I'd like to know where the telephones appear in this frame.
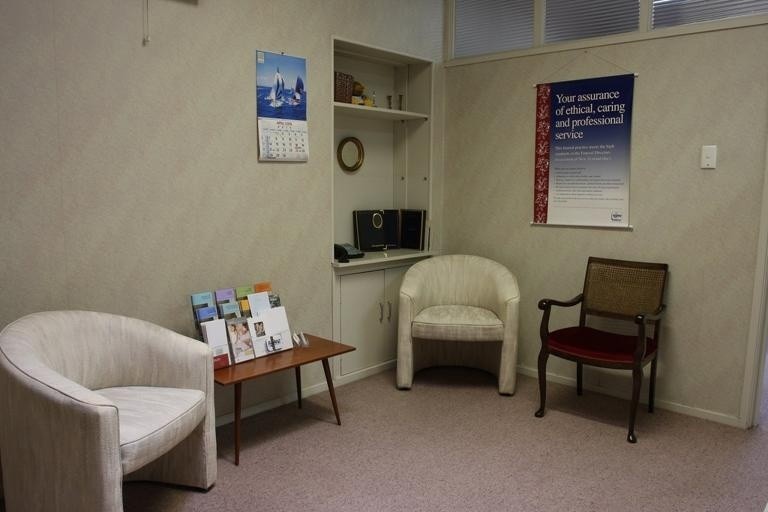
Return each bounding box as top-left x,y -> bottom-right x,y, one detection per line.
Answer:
333,242 -> 364,259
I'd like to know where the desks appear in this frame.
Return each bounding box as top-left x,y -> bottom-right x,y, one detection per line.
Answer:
214,334 -> 357,467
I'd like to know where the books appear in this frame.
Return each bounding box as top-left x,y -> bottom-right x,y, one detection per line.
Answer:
191,281 -> 294,372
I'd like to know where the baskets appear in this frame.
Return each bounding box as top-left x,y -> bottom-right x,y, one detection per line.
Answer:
334,71 -> 352,104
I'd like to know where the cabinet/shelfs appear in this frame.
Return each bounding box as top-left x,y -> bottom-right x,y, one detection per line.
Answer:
331,34 -> 434,385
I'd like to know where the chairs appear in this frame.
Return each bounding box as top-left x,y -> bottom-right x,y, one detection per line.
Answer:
535,254 -> 669,443
395,253 -> 520,395
0,310 -> 218,512
